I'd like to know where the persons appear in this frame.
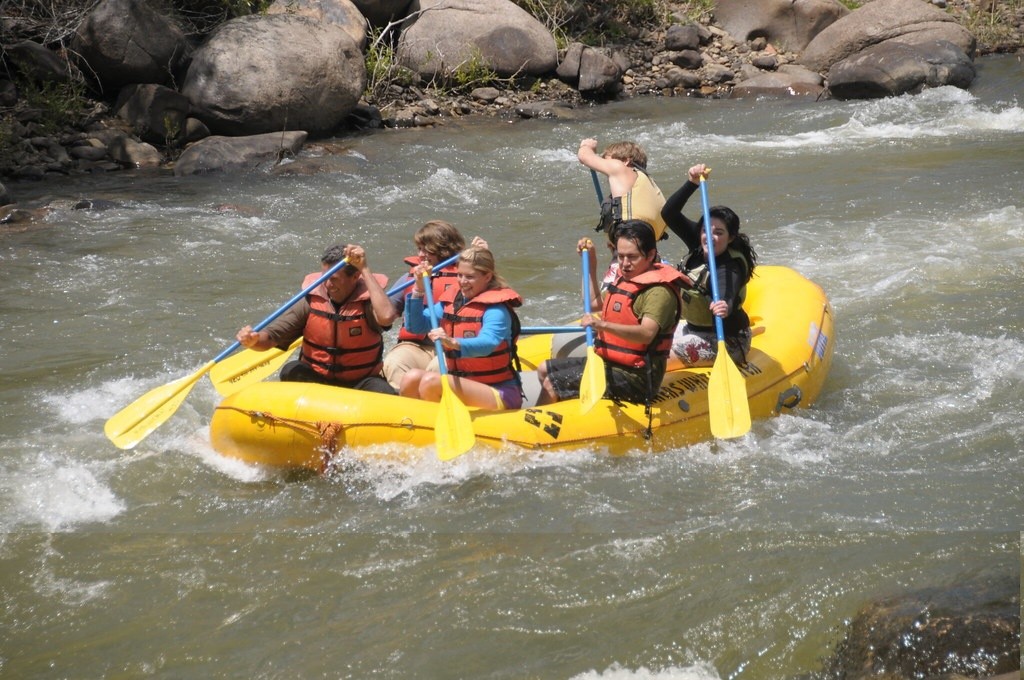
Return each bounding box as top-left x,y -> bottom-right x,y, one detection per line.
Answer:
400,246 -> 523,410
578,138 -> 667,292
236,243 -> 396,394
380,219 -> 488,392
535,217 -> 694,407
661,164 -> 760,372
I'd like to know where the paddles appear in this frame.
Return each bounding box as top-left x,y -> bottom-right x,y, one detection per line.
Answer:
588,136 -> 606,207
208,250 -> 463,398
103,254 -> 351,451
700,169 -> 754,440
515,324 -> 588,336
578,239 -> 608,417
421,269 -> 476,462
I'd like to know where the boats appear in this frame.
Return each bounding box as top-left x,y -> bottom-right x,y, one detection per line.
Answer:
210,265 -> 834,476
